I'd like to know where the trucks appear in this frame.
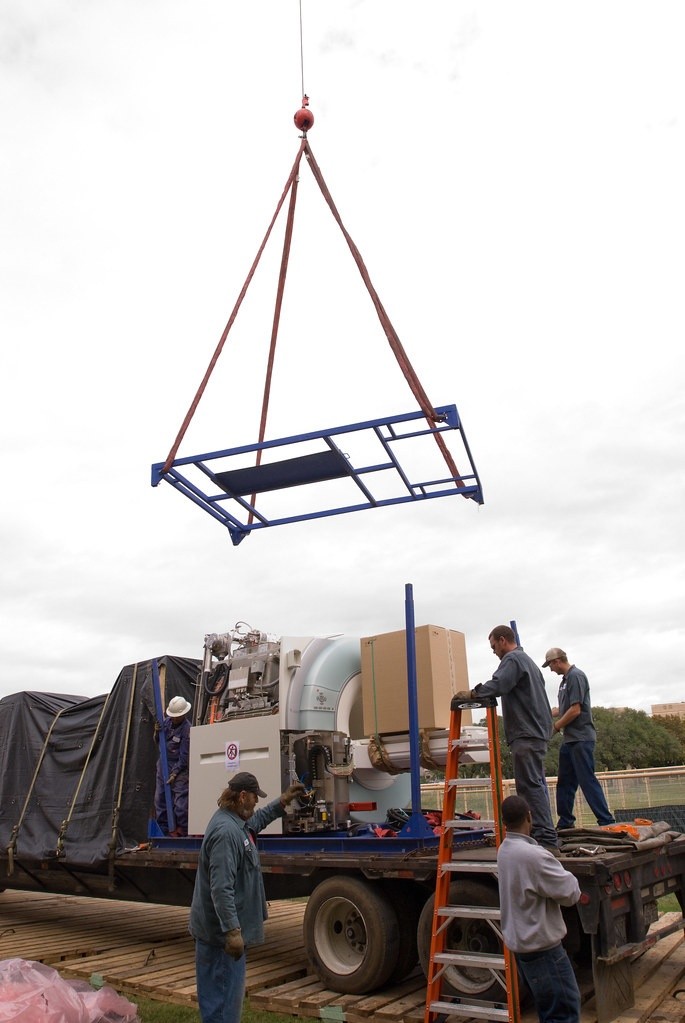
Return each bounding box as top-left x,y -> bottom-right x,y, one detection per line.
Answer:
1,622 -> 684,996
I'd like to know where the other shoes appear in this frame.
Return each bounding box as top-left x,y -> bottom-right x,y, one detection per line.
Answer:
544,847 -> 562,857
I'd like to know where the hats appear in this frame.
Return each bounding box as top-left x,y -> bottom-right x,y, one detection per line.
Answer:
228,771 -> 267,799
542,648 -> 566,668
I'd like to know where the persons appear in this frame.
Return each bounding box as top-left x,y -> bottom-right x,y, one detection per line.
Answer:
153,696 -> 191,836
542,648 -> 615,829
452,625 -> 561,857
496,795 -> 581,1023
187,773 -> 304,1023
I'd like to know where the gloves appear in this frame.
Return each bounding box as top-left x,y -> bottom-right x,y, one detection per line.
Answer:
453,690 -> 473,700
154,721 -> 162,735
166,773 -> 177,784
551,723 -> 559,739
225,928 -> 244,962
279,783 -> 305,807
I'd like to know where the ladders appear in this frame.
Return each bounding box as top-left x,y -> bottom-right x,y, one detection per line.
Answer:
422,694 -> 525,1023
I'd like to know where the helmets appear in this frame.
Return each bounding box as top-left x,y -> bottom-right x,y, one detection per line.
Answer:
165,696 -> 191,717
